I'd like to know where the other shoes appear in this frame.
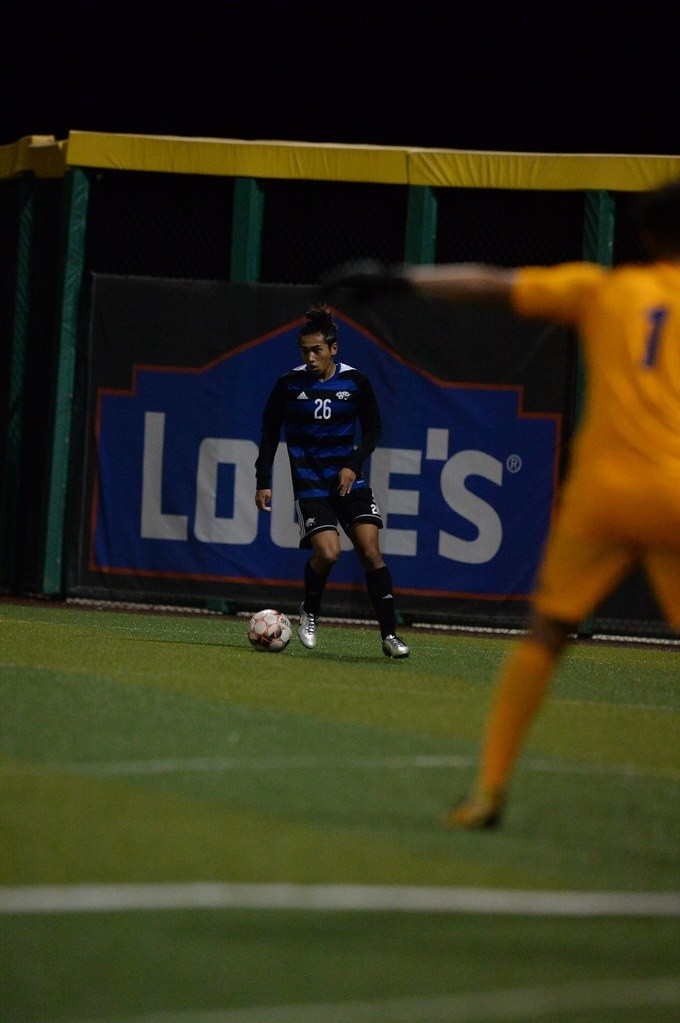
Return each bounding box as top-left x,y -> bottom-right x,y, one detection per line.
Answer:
444,779 -> 507,830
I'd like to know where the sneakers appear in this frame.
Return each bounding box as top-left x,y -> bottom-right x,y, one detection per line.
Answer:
382,633 -> 410,658
298,601 -> 319,649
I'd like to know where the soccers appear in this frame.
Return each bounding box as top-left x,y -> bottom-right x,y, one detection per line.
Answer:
246,607 -> 294,652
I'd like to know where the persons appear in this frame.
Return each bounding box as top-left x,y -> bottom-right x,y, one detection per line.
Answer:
407,182 -> 680,833
253,307 -> 410,659
